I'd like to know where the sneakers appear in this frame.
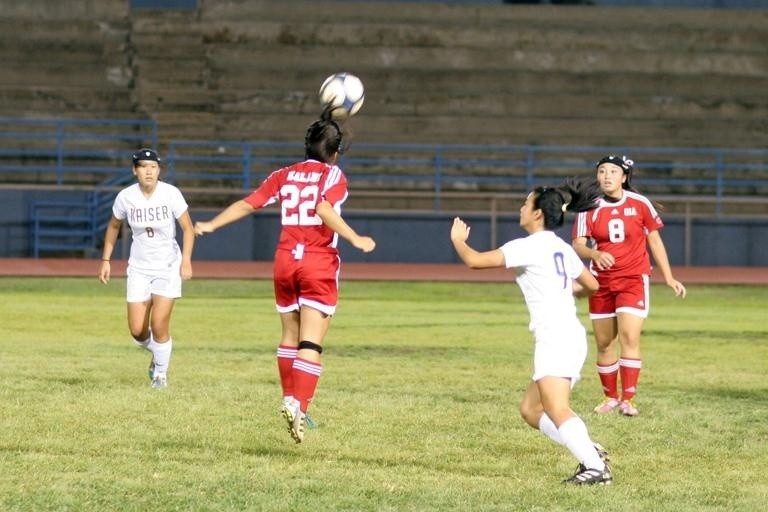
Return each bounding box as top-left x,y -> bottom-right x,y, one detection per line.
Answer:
593,396 -> 620,415
560,444 -> 613,488
149,353 -> 166,389
618,399 -> 639,417
281,398 -> 316,444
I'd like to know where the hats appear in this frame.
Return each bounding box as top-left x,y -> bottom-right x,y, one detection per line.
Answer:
596,154 -> 630,172
131,148 -> 161,163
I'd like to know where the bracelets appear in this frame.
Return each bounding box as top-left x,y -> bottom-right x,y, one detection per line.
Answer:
100,257 -> 112,263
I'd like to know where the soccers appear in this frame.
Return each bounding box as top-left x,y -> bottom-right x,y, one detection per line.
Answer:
319,72 -> 364,118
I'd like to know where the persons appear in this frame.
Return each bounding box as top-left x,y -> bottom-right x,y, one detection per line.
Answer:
98,147 -> 197,384
450,172 -> 612,484
570,153 -> 689,414
190,105 -> 375,446
